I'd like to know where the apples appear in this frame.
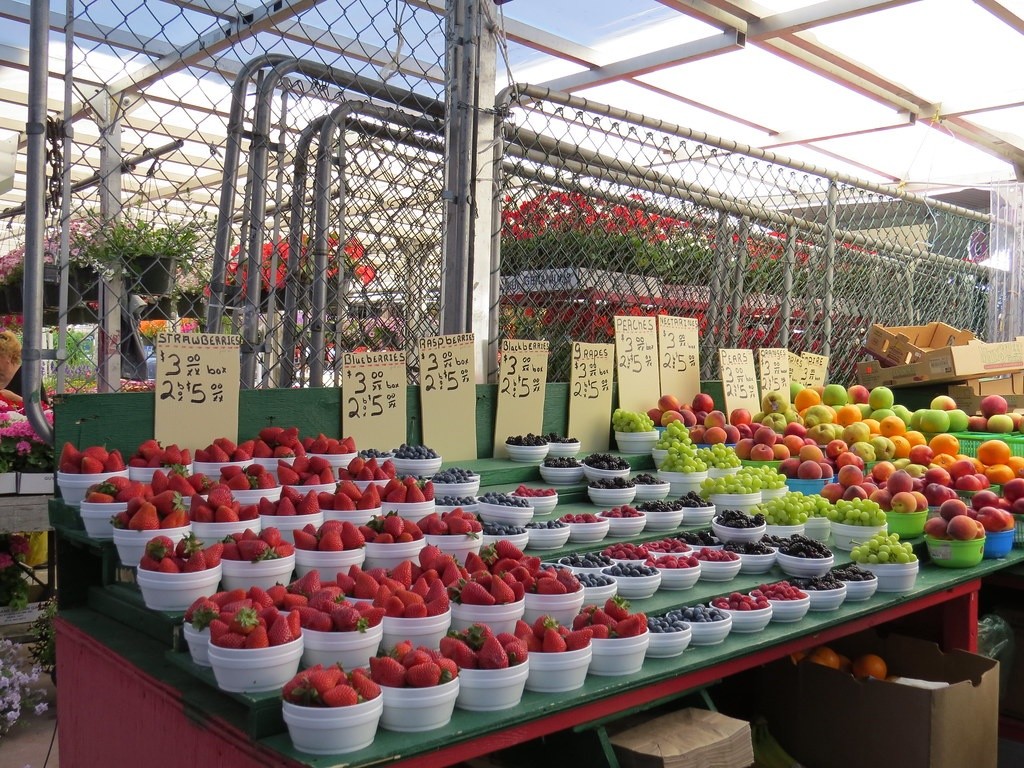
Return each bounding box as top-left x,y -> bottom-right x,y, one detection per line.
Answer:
787,382 -> 1024,434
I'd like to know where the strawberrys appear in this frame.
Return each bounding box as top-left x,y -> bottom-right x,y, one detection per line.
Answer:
59,425 -> 647,708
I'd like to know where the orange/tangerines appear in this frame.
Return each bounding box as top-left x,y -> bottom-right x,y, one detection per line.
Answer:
793,389 -> 1024,483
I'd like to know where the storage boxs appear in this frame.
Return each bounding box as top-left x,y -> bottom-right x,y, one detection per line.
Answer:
499,266 -> 864,311
766,632 -> 1001,767
857,322 -> 1024,415
0,582 -> 49,627
0,472 -> 54,494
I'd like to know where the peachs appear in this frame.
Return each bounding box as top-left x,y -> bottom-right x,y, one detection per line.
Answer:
735,422 -> 986,540
963,478 -> 1024,533
645,394 -> 763,445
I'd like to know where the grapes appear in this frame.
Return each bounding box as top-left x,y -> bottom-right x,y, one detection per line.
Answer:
612,409 -> 918,565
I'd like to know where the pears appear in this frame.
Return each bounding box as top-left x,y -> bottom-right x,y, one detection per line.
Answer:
752,391 -> 944,478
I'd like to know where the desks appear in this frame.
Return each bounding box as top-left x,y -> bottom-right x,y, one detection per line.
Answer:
49,381 -> 1024,768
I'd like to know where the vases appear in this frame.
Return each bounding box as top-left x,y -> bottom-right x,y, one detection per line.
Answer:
143,345 -> 155,379
0,254 -> 381,324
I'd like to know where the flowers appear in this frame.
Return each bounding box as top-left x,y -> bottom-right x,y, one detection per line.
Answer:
497,189 -> 877,298
0,532 -> 49,611
0,394 -> 55,477
0,198 -> 378,300
138,311 -> 406,351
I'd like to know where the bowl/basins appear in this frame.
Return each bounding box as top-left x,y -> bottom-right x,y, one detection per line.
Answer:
588,481 -> 671,507
57,451 -> 649,754
654,422 -> 1016,569
507,491 -> 558,515
558,515 -> 878,658
615,428 -> 919,593
556,505 -> 716,543
504,441 -> 580,461
539,461 -> 631,485
